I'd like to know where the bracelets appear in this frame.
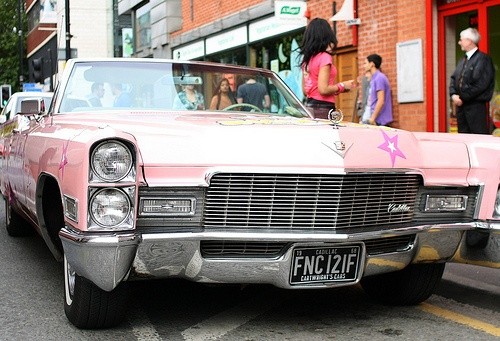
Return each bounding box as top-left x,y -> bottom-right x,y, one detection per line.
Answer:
334,82 -> 345,95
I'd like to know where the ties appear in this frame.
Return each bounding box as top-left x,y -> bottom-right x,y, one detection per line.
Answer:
457,55 -> 468,92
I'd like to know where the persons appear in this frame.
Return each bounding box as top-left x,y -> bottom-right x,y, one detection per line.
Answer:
363,53 -> 393,126
173,72 -> 206,110
235,75 -> 270,112
359,71 -> 373,125
449,27 -> 496,135
209,78 -> 238,111
110,83 -> 137,108
291,17 -> 356,120
85,82 -> 106,108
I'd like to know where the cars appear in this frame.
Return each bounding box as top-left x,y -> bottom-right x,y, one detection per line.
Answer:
1,92 -> 93,126
0,57 -> 500,330
